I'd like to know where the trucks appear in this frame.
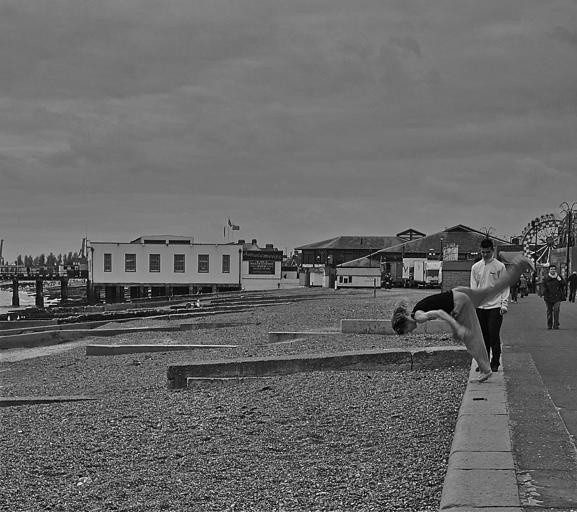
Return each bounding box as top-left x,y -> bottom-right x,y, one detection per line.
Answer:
402,257 -> 442,289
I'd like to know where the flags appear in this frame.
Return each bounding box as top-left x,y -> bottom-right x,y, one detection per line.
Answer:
223,217 -> 233,238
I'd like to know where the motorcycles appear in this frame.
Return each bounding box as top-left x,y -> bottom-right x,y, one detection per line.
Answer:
384,274 -> 393,289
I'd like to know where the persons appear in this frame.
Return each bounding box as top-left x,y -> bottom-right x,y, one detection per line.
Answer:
511,264 -> 577,305
470,239 -> 511,375
381,270 -> 393,290
390,255 -> 537,383
539,265 -> 566,330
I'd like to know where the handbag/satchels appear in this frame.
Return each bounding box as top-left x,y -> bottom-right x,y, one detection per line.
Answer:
560,285 -> 567,301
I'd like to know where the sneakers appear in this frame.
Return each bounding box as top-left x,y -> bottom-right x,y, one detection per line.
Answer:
474,363 -> 499,382
517,254 -> 536,272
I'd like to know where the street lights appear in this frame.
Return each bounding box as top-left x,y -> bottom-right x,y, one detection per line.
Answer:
559,201 -> 577,302
86,246 -> 95,300
